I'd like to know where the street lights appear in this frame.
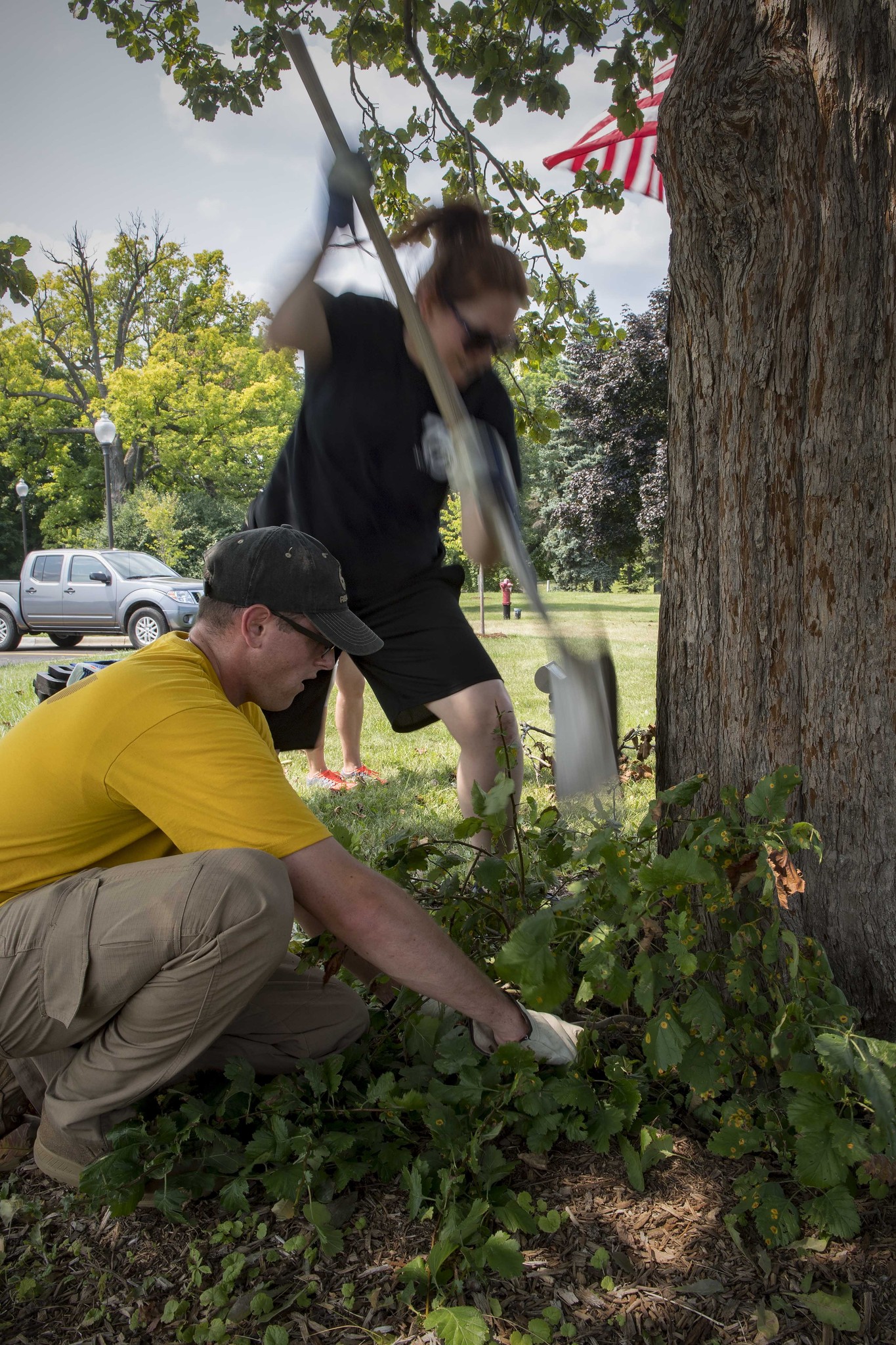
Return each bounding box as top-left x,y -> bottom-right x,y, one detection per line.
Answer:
94,409 -> 122,549
15,477 -> 30,559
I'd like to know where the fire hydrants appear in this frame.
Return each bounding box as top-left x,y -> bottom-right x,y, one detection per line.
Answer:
498,579 -> 514,620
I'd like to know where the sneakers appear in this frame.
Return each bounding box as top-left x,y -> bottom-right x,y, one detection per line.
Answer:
304,770 -> 356,792
342,767 -> 389,788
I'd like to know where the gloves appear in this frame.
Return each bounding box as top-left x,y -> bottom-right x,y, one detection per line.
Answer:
466,989 -> 586,1064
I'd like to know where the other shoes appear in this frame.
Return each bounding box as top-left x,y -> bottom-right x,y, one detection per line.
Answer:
33,1104 -> 113,1183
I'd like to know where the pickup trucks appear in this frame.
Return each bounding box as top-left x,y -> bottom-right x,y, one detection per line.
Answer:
0,548 -> 205,650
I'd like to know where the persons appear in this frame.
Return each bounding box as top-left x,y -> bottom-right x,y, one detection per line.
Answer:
0,524 -> 583,1188
255,152 -> 527,873
302,649 -> 389,790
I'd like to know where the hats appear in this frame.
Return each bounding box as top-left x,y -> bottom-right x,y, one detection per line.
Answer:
204,523 -> 385,656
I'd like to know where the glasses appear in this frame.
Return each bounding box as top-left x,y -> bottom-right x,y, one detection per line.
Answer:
438,288 -> 520,354
266,607 -> 336,659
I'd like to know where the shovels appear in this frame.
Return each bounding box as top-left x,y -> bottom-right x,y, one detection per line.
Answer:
275,23 -> 624,807
